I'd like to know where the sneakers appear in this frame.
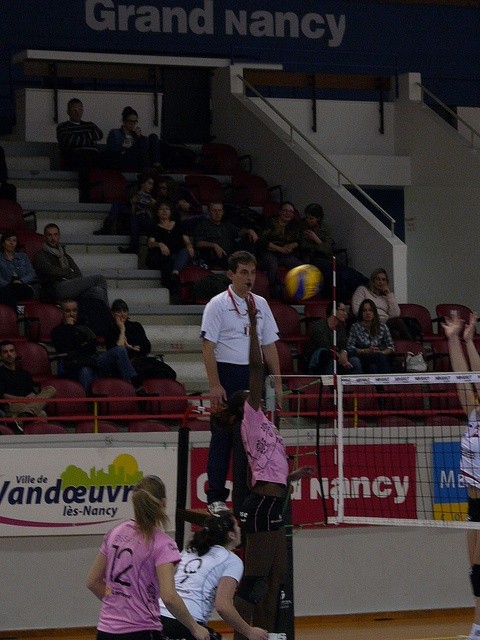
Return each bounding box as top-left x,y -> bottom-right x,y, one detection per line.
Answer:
13,414 -> 27,432
207,500 -> 230,518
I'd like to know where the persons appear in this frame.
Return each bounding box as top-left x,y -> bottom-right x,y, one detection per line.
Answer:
0,231 -> 39,310
145,202 -> 194,277
228,294 -> 287,639
259,201 -> 304,288
32,223 -> 108,306
182,200 -> 258,266
118,177 -> 158,251
344,299 -> 397,374
301,300 -> 354,375
84,474 -> 212,639
352,268 -> 399,327
437,307 -> 480,638
303,203 -> 335,259
0,339 -> 56,433
201,250 -> 285,516
155,510 -> 271,639
56,97 -> 104,171
107,298 -> 177,385
107,106 -> 163,176
50,298 -> 159,398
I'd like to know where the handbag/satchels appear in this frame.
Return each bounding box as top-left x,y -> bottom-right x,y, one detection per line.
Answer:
0,277 -> 34,301
405,351 -> 428,372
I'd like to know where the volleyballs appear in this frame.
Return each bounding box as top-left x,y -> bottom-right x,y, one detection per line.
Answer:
286,265 -> 318,300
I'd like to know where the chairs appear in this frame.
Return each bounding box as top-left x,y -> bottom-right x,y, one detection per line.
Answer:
396,340 -> 425,373
436,303 -> 480,344
90,378 -> 155,416
22,423 -> 69,435
144,379 -> 203,417
275,341 -> 294,376
39,379 -> 87,415
252,271 -> 268,298
399,303 -> 445,344
15,341 -> 58,384
19,231 -> 45,258
432,341 -> 453,371
383,383 -> 422,420
269,301 -> 303,343
128,421 -> 168,432
290,377 -> 324,413
0,424 -> 13,435
411,383 -> 469,418
26,301 -> 62,340
0,305 -> 26,341
338,382 -> 381,418
375,415 -> 416,426
0,200 -> 24,234
186,421 -> 209,432
329,417 -> 367,429
424,415 -> 464,425
76,421 -> 119,433
304,301 -> 327,328
